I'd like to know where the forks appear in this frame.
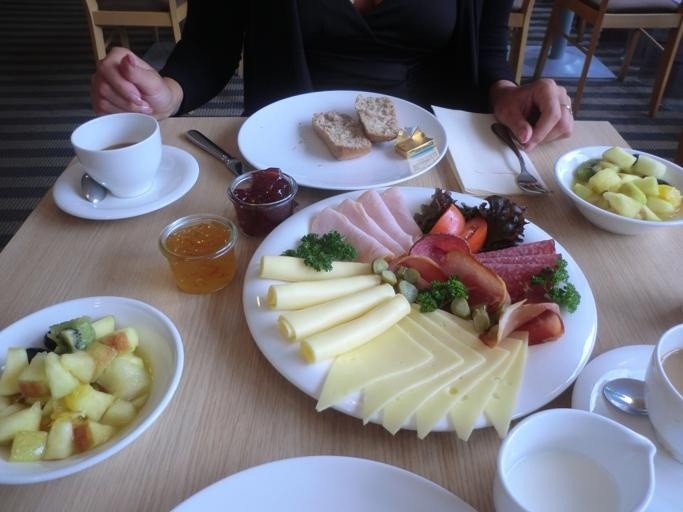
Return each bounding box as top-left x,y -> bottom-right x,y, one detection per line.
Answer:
491,122 -> 554,195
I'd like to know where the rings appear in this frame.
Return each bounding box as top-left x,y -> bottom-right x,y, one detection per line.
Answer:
561,102 -> 574,116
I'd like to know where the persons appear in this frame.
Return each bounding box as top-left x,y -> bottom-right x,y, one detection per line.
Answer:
88,0 -> 576,155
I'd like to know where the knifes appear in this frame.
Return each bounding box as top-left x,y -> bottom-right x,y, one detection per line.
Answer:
184,129 -> 300,210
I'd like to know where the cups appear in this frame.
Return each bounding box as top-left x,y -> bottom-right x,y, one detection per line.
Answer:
224,170 -> 298,237
158,213 -> 237,294
69,112 -> 161,199
491,409 -> 657,511
642,323 -> 683,465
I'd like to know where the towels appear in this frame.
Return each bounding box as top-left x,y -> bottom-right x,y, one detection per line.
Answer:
431,105 -> 548,201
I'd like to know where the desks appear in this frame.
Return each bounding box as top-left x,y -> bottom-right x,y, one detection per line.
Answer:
506,2 -> 617,83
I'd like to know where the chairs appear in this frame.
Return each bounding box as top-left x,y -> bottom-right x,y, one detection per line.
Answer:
470,1 -> 533,88
79,0 -> 187,76
540,1 -> 682,120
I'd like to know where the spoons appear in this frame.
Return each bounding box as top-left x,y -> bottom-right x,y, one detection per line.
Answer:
80,173 -> 107,203
602,377 -> 649,416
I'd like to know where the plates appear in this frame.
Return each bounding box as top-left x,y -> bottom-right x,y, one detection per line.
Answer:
0,294 -> 184,486
51,144 -> 199,220
240,186 -> 598,432
571,343 -> 683,510
236,90 -> 449,190
167,455 -> 475,511
552,145 -> 683,236
